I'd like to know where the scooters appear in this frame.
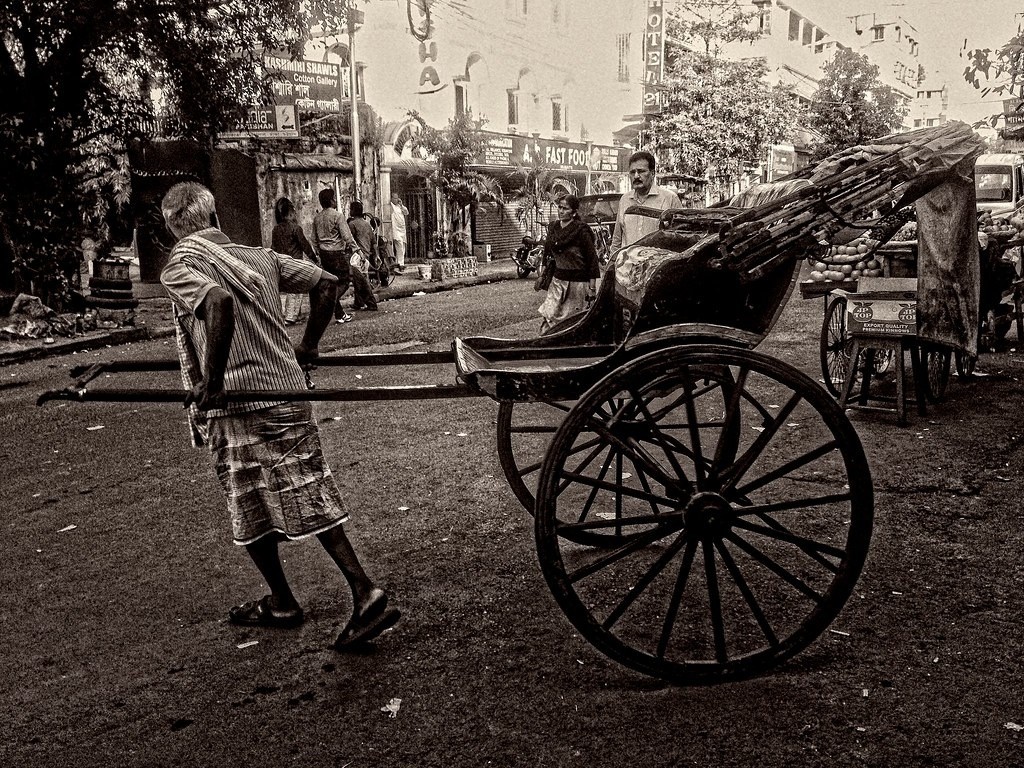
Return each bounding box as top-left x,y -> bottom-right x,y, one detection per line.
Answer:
588,213 -> 611,265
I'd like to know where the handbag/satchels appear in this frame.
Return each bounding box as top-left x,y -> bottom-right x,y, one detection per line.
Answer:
540,254 -> 556,291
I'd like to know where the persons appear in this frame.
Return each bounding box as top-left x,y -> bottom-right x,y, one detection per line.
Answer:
159,181 -> 390,648
272,197 -> 319,326
537,194 -> 601,330
390,192 -> 409,267
310,189 -> 391,325
608,153 -> 684,258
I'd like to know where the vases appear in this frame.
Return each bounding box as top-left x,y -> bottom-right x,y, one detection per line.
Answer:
418,265 -> 433,280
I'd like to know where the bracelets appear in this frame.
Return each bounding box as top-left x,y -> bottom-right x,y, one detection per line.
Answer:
589,286 -> 596,290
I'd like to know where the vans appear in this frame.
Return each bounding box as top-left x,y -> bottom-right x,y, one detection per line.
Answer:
974,153 -> 1024,215
578,193 -> 624,236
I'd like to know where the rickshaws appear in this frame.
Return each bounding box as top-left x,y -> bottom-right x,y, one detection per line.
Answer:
38,121 -> 988,687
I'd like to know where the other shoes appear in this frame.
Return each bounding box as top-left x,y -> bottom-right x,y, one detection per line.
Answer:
285,313 -> 307,324
361,306 -> 378,311
348,304 -> 361,310
399,267 -> 404,272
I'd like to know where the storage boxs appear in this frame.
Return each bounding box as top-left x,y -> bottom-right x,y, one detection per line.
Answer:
474,245 -> 492,262
835,276 -> 919,336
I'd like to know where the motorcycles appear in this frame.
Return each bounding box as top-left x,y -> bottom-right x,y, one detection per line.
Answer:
509,219 -> 551,278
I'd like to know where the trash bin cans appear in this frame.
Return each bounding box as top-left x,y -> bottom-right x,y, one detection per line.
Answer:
84,260 -> 139,324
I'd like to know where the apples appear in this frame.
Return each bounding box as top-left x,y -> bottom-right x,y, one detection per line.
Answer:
811,244 -> 883,283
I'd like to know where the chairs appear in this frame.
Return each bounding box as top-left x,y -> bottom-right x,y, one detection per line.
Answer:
615,177 -> 816,338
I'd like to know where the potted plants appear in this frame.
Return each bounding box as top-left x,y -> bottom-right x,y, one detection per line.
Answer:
425,231 -> 477,280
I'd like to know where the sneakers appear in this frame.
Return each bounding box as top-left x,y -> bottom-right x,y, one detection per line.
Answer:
335,313 -> 352,323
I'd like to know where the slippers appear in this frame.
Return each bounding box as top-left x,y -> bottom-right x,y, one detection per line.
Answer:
230,595 -> 305,628
334,606 -> 402,652
